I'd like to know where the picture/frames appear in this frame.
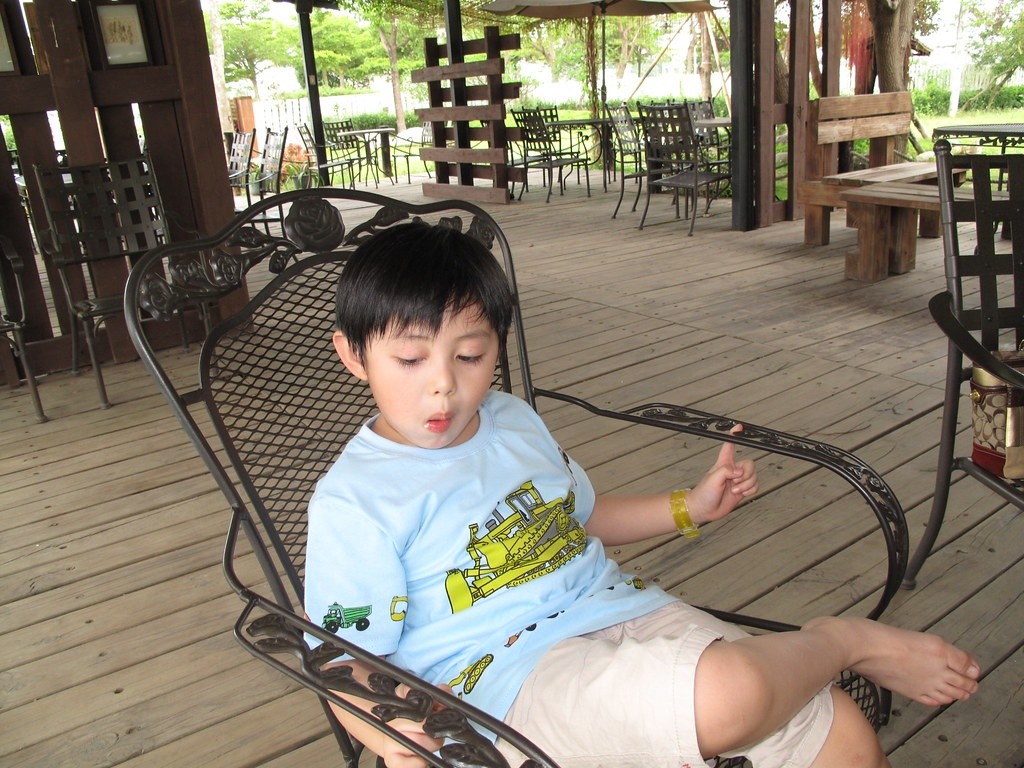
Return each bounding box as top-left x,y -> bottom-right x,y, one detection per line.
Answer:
88,0 -> 155,70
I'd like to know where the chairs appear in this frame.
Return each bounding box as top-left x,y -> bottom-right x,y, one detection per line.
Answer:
0,93 -> 430,427
481,100 -> 731,236
122,185 -> 909,768
898,134 -> 1024,591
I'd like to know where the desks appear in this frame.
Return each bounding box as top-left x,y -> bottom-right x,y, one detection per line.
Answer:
337,127 -> 395,190
549,118 -> 612,196
695,117 -> 731,217
935,124 -> 1024,242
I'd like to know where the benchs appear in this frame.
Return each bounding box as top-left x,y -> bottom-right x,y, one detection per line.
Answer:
799,91 -> 1010,283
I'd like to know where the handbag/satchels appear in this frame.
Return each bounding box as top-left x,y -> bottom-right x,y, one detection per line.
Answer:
969,340 -> 1024,484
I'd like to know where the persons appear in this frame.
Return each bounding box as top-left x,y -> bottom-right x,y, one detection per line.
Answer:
299,223 -> 982,767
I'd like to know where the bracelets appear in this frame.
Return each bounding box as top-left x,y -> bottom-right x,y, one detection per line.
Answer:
669,481 -> 700,540
